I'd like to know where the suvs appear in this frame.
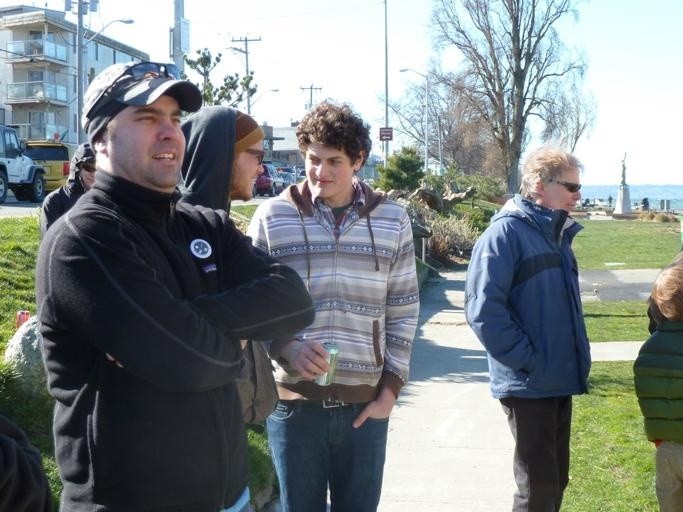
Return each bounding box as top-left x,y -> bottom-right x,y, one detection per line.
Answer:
12,136 -> 80,203
0,123 -> 46,205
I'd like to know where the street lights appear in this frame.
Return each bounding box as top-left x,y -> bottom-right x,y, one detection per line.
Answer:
399,68 -> 429,173
247,88 -> 279,117
64,0 -> 134,146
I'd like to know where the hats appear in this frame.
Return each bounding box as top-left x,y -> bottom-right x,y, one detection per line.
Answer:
233,110 -> 265,154
82,61 -> 202,155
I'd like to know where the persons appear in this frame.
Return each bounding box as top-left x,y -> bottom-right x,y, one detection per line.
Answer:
634,252 -> 683,512
41,143 -> 96,237
36,60 -> 315,512
244,100 -> 420,512
464,148 -> 591,512
176,106 -> 279,431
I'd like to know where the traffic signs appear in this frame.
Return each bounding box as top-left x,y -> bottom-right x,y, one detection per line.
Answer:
378,127 -> 394,141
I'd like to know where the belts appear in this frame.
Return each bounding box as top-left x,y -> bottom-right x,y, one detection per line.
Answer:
303,400 -> 348,409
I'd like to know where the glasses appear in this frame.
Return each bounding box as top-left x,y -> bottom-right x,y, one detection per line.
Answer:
246,149 -> 264,164
548,179 -> 581,192
85,62 -> 179,118
82,163 -> 95,173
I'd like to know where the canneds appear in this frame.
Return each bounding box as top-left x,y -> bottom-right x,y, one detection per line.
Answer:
16,310 -> 30,330
314,342 -> 338,386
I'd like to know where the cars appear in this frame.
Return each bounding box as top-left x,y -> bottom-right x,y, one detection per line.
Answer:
254,163 -> 307,199
